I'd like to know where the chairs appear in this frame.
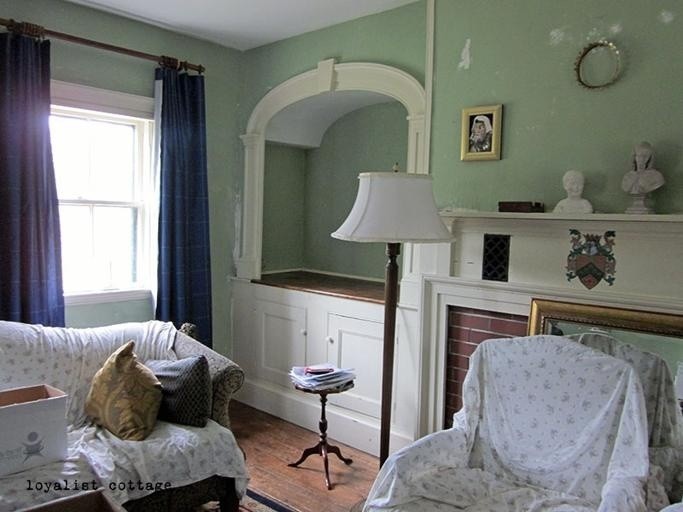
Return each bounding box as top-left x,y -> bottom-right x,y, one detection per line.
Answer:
567,335 -> 683,512
363,333 -> 650,510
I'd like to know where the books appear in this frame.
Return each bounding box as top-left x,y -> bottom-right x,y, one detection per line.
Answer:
288,361 -> 356,390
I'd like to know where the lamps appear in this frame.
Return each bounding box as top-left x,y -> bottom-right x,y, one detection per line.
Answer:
331,164 -> 456,469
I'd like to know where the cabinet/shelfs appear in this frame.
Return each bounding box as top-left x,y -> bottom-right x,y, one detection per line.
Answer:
229,280 -> 398,432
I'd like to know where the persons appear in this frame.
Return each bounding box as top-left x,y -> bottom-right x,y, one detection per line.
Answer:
470,116 -> 491,152
620,142 -> 666,195
551,171 -> 594,213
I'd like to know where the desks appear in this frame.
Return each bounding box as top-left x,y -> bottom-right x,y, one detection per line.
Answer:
287,378 -> 354,488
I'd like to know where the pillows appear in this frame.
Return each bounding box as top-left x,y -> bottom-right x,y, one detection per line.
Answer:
81,340 -> 163,441
143,352 -> 212,428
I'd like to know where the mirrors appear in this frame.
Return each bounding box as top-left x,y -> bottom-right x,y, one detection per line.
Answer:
527,296 -> 683,512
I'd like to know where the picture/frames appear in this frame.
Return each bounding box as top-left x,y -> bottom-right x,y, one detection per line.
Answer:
459,103 -> 504,162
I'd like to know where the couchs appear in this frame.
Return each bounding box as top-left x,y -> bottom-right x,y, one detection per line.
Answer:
0,317 -> 248,512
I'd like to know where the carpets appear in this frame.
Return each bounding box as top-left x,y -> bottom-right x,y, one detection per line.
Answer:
185,484 -> 302,512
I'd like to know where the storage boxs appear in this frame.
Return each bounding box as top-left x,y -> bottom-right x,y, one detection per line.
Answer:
0,380 -> 69,474
